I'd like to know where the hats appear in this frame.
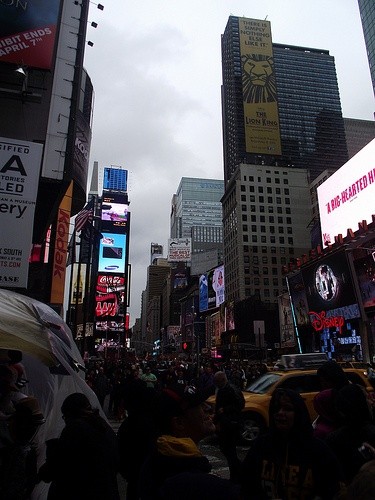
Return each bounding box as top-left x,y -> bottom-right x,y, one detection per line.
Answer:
15,397 -> 43,417
314,389 -> 332,419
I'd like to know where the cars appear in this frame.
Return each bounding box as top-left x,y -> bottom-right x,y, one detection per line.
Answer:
200,368 -> 375,448
338,362 -> 375,380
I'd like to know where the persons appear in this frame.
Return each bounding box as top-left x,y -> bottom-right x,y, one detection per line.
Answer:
39,392 -> 121,500
93,350 -> 375,500
